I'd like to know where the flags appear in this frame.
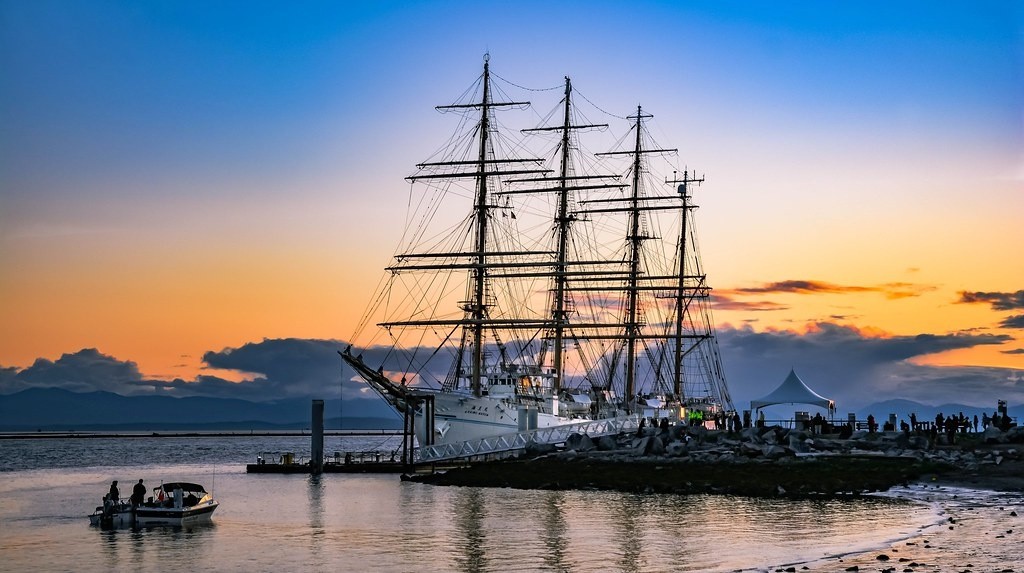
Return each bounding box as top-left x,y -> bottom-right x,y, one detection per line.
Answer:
158,486 -> 164,501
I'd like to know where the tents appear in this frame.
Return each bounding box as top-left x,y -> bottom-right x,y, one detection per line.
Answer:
747,367 -> 836,429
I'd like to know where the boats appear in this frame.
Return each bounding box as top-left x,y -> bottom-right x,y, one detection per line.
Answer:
88,504 -> 135,528
136,480 -> 219,525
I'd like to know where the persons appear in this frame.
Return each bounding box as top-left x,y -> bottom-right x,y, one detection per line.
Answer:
567,396 -> 1016,443
133,479 -> 146,506
173,484 -> 184,509
110,481 -> 119,506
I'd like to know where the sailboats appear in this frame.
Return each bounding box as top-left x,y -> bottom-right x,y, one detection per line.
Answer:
336,52 -> 743,459
